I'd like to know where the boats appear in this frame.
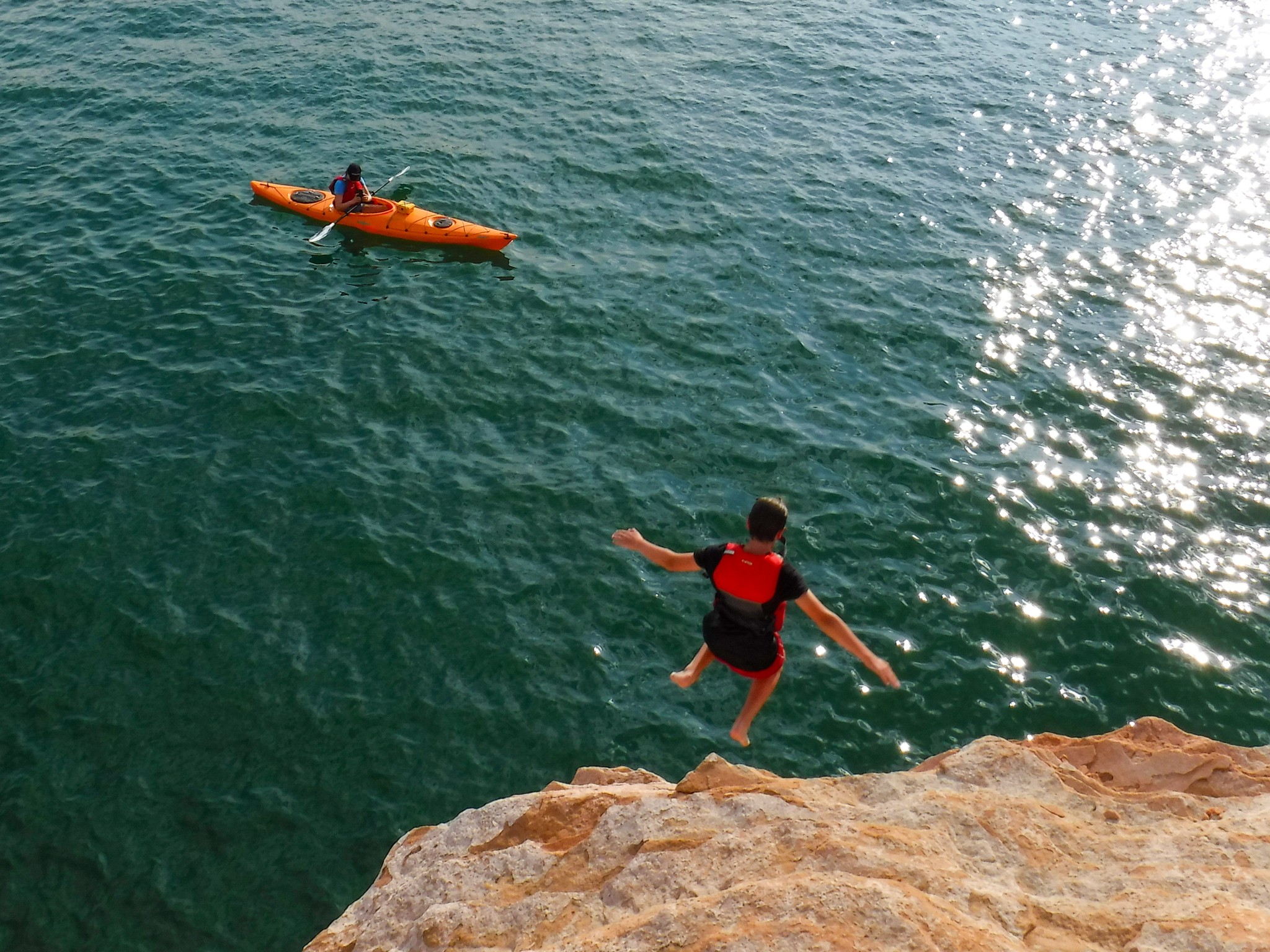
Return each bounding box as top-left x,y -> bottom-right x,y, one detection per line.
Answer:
250,180 -> 518,251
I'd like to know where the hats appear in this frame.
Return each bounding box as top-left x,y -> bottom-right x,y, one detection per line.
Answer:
347,163 -> 362,181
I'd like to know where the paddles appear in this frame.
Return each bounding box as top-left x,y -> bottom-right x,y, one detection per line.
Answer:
308,165 -> 411,243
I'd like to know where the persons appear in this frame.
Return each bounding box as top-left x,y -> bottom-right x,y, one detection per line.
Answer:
333,164 -> 372,214
612,496 -> 901,746
